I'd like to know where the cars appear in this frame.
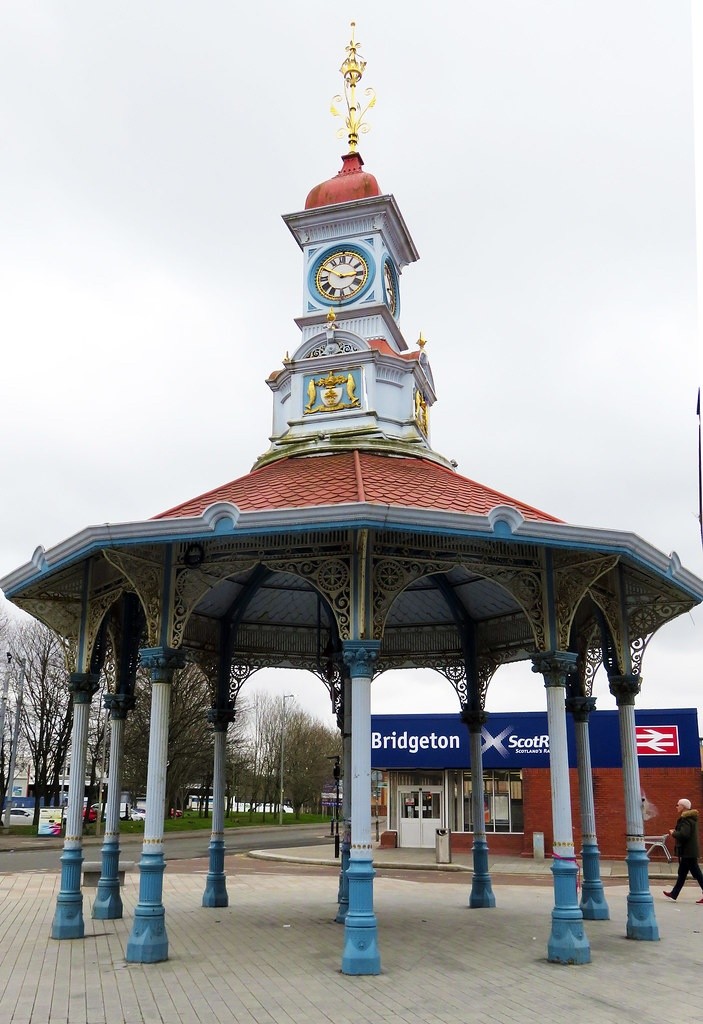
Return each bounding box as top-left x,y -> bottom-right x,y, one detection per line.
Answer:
82,806 -> 96,824
130,809 -> 145,821
1,808 -> 55,825
171,808 -> 182,818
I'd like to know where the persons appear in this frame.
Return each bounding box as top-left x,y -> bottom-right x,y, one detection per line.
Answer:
663,798 -> 703,906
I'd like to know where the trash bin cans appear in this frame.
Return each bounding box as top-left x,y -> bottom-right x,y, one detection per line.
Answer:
435,828 -> 451,863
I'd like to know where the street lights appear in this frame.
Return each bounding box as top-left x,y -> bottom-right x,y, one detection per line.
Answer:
279,694 -> 294,826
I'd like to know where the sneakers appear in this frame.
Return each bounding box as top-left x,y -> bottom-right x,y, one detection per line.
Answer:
662,891 -> 703,904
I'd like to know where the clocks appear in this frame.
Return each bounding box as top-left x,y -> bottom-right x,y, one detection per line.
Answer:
307,242 -> 378,307
